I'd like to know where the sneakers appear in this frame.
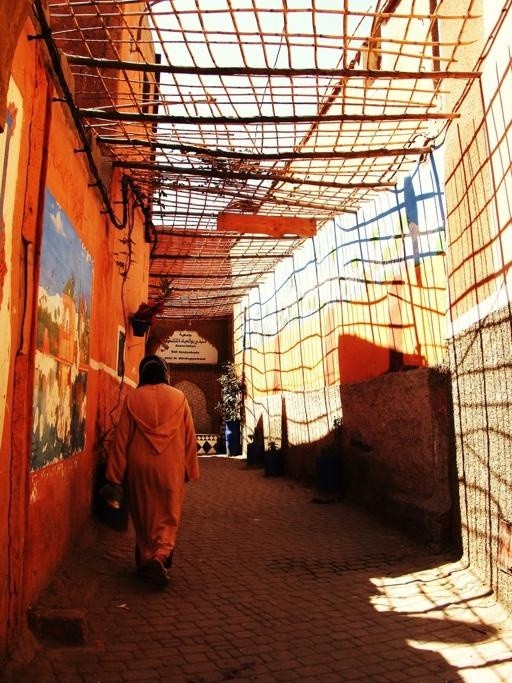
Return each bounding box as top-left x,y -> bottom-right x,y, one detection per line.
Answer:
136,558 -> 171,586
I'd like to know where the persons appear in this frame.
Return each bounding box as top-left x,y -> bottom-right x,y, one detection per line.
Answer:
105,354 -> 199,584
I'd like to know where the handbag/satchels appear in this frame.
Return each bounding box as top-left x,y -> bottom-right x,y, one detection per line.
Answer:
100,482 -> 123,509
